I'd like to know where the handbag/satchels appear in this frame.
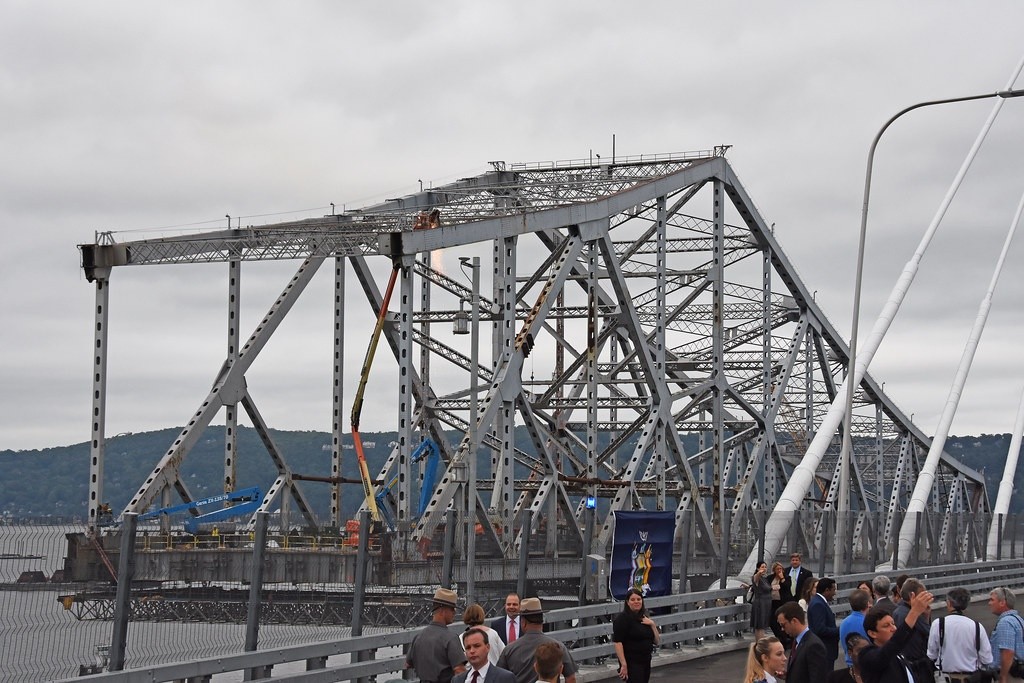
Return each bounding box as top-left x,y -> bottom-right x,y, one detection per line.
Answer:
745,585 -> 755,604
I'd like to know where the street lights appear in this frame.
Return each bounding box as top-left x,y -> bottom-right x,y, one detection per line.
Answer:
829,89 -> 1024,586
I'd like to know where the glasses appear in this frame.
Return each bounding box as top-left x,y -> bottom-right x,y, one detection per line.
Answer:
780,620 -> 789,630
1000,585 -> 1009,607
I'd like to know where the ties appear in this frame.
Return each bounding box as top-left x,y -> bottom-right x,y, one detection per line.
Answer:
470,671 -> 481,683
790,569 -> 797,597
789,640 -> 798,665
508,619 -> 517,644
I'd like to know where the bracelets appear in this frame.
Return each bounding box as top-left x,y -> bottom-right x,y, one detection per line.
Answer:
780,577 -> 785,580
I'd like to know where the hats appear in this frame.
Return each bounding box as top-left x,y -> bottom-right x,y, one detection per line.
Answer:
424,588 -> 465,609
513,597 -> 550,616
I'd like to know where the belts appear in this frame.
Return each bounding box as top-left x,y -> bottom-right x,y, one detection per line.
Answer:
946,672 -> 975,674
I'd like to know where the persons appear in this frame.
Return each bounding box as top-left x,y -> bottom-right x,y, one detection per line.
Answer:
496,597 -> 578,683
491,592 -> 525,646
404,586 -> 468,683
926,586 -> 994,683
459,602 -> 507,667
774,601 -> 828,683
450,627 -> 519,683
744,635 -> 787,683
611,587 -> 661,683
740,551 -> 1024,683
989,585 -> 1024,683
532,642 -> 565,683
858,590 -> 935,683
